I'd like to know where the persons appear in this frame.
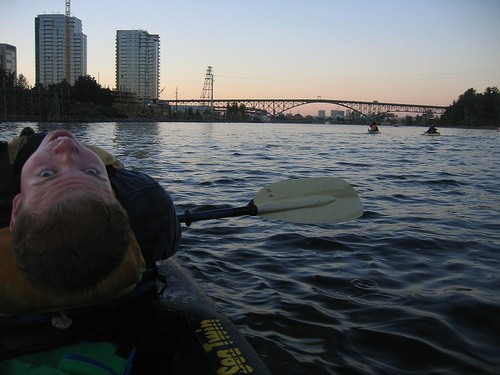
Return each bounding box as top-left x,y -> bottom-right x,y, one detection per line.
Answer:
0,127 -> 182,292
425,124 -> 436,134
370,122 -> 378,131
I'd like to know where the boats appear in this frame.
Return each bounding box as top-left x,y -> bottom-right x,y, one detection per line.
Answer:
422,131 -> 441,137
368,126 -> 382,135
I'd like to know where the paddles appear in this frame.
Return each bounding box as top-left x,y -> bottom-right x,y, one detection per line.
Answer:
177,178 -> 365,227
